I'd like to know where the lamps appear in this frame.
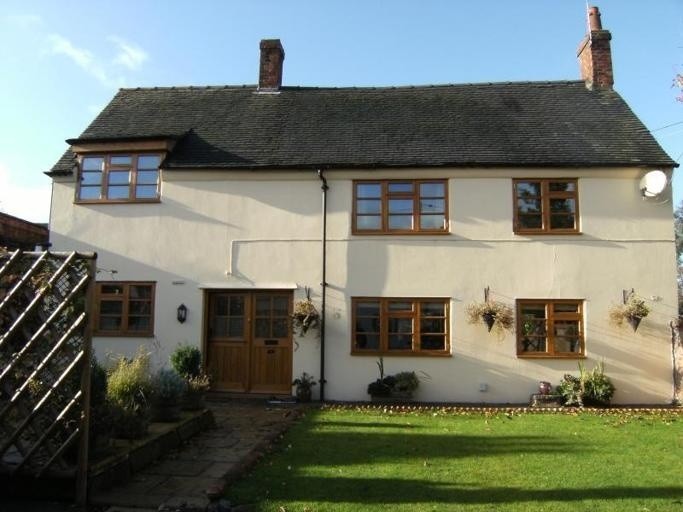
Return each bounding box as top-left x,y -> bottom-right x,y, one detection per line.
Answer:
174,301 -> 190,327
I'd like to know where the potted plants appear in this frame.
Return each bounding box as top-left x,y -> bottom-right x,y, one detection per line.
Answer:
291,372 -> 317,406
61,349 -> 211,468
467,288 -> 513,333
292,288 -> 321,337
366,358 -> 420,406
607,290 -> 653,332
